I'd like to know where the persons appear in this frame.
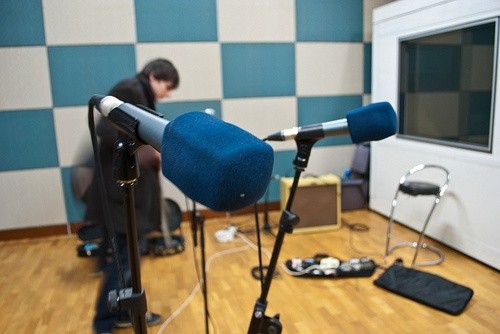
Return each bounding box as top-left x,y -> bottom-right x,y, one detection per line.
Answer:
84,58 -> 179,334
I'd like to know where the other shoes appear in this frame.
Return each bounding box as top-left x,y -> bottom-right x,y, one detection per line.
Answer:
115,311 -> 161,327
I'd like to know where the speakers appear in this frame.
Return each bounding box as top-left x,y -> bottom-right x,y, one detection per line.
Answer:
279,174 -> 342,235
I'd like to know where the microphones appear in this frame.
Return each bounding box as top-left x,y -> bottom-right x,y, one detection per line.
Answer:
91,93 -> 274,212
267,101 -> 398,144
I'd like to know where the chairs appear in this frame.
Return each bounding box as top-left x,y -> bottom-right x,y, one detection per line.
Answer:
342,143 -> 370,209
385,163 -> 451,268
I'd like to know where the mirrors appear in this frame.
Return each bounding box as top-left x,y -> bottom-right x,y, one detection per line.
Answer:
396,15 -> 500,153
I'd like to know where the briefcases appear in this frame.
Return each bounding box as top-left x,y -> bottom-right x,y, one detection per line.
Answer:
281,174 -> 342,234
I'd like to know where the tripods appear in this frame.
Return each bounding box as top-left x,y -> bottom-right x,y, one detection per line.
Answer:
236,182 -> 278,238
